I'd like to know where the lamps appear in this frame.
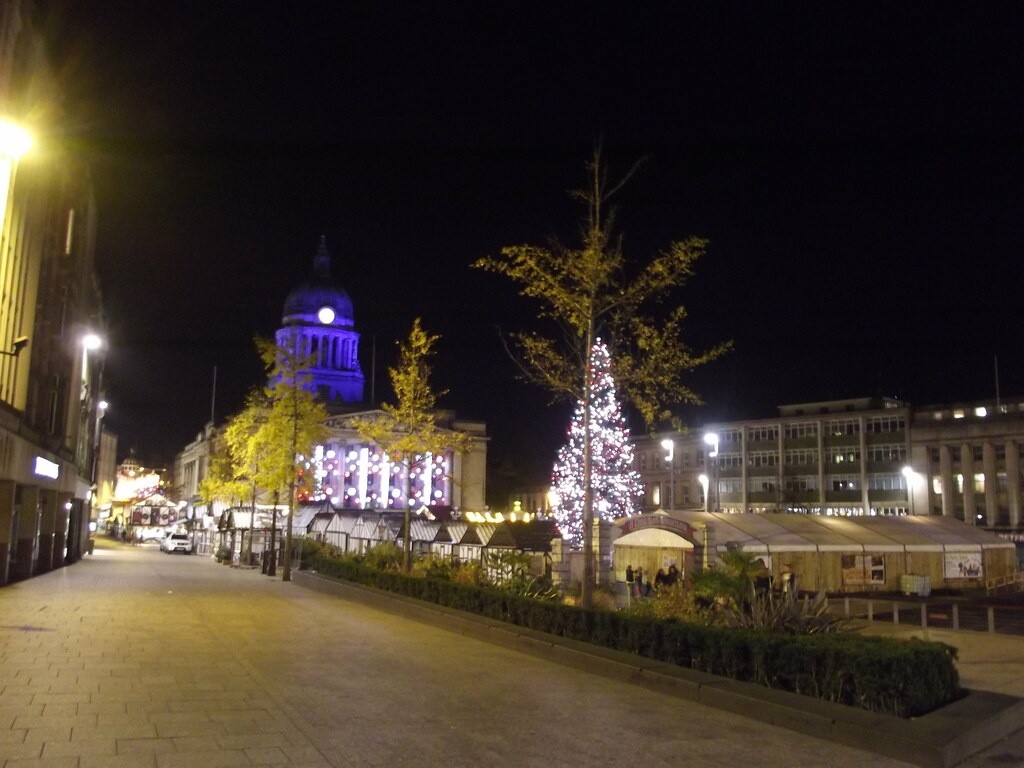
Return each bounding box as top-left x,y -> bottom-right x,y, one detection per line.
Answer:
0,336 -> 30,357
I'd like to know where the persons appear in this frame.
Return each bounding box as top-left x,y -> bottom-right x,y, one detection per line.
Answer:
655,566 -> 679,589
626,566 -> 654,597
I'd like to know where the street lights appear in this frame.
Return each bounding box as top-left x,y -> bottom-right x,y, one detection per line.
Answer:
661,438 -> 675,511
699,475 -> 710,513
704,432 -> 721,512
901,466 -> 915,516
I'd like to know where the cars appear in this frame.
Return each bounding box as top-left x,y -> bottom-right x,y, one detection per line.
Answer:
159,529 -> 193,554
